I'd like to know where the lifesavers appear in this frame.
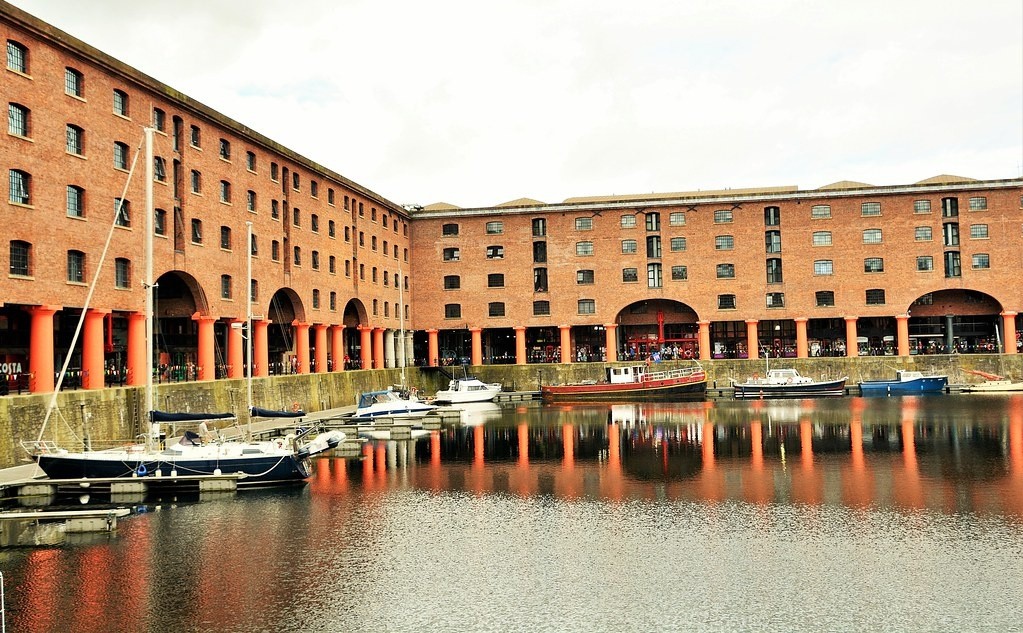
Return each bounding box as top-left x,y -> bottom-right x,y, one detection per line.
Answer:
753,375 -> 759,380
787,377 -> 793,383
292,402 -> 299,412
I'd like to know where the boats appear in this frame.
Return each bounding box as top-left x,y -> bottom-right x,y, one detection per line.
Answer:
435,377 -> 502,404
352,390 -> 441,428
959,378 -> 1023,392
541,358 -> 708,402
733,353 -> 849,400
857,372 -> 949,392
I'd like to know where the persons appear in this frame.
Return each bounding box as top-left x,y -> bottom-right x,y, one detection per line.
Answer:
327,352 -> 331,372
839,343 -> 844,356
343,353 -> 350,371
951,338 -> 958,353
775,343 -> 781,358
815,343 -> 821,357
627,348 -> 635,360
108,363 -> 115,370
355,354 -> 360,361
661,345 -> 683,360
686,350 -> 691,359
198,421 -> 212,444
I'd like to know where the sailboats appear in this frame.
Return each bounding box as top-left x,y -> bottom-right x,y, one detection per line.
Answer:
16,124 -> 349,486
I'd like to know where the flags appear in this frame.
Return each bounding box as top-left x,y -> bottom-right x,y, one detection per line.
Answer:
652,352 -> 661,363
645,357 -> 651,366
